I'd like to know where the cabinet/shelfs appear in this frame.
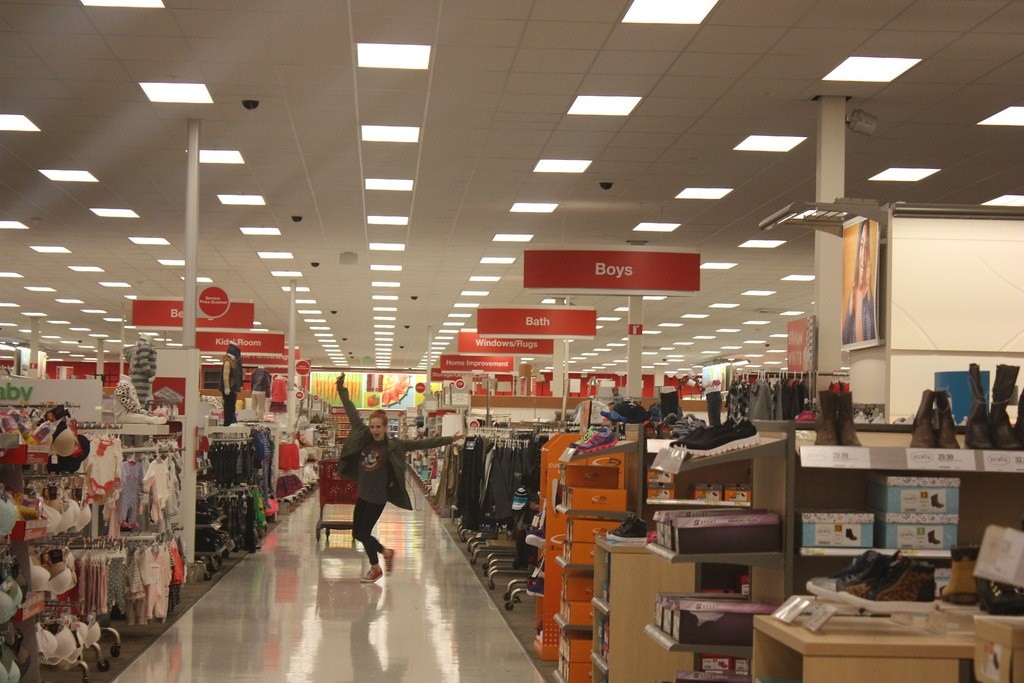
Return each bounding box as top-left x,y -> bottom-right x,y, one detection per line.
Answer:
552,438 -> 639,682
195,467 -> 230,558
794,443 -> 1024,563
645,432 -> 785,683
330,407 -> 351,439
362,416 -> 399,438
593,535 -> 696,683
750,614 -> 973,683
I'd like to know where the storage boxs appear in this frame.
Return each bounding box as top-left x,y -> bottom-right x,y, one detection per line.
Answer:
972,614 -> 1024,683
557,631 -> 591,682
562,518 -> 620,564
560,571 -> 595,625
559,463 -> 627,510
651,508 -> 785,553
653,589 -> 781,645
664,656 -> 751,683
800,476 -> 960,550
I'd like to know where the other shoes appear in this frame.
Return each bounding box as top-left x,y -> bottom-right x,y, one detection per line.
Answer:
831,545 -> 1024,616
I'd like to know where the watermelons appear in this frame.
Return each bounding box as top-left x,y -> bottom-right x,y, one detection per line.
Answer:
382,375 -> 411,406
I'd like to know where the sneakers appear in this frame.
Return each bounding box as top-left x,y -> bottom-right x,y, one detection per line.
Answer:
657,422 -> 672,439
360,566 -> 383,583
570,425 -> 603,448
383,549 -> 393,575
576,430 -> 617,452
672,414 -> 696,437
670,414 -> 759,458
644,421 -> 656,439
527,577 -> 544,597
525,525 -> 546,550
606,513 -> 647,543
681,416 -> 706,435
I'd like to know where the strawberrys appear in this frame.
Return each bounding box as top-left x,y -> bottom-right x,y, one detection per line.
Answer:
367,394 -> 377,406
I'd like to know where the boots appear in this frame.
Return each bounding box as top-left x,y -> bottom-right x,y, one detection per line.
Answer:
815,391 -> 837,446
706,391 -> 722,425
936,391 -> 960,449
837,389 -> 861,447
661,391 -> 679,418
965,363 -> 993,449
910,390 -> 934,448
987,365 -> 1024,450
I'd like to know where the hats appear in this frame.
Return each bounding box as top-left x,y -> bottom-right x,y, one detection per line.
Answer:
512,488 -> 529,511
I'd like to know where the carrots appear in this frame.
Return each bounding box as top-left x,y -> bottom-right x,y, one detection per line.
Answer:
315,379 -> 361,401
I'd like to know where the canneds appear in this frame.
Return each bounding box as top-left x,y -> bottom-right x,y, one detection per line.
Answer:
329,417 -> 350,436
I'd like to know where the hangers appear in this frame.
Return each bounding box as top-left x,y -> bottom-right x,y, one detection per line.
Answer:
205,435 -> 254,452
531,419 -> 584,435
459,427 -> 528,451
729,366 -> 813,388
216,485 -> 261,506
0,400 -> 185,658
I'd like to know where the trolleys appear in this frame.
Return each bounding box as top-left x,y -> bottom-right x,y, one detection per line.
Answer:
316,443 -> 360,542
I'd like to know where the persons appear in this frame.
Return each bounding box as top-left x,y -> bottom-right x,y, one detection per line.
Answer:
843,220 -> 875,345
660,386 -> 678,419
113,374 -> 167,424
250,366 -> 270,418
706,387 -> 722,426
128,333 -> 157,411
271,374 -> 287,403
219,341 -> 241,426
335,373 -> 466,583
